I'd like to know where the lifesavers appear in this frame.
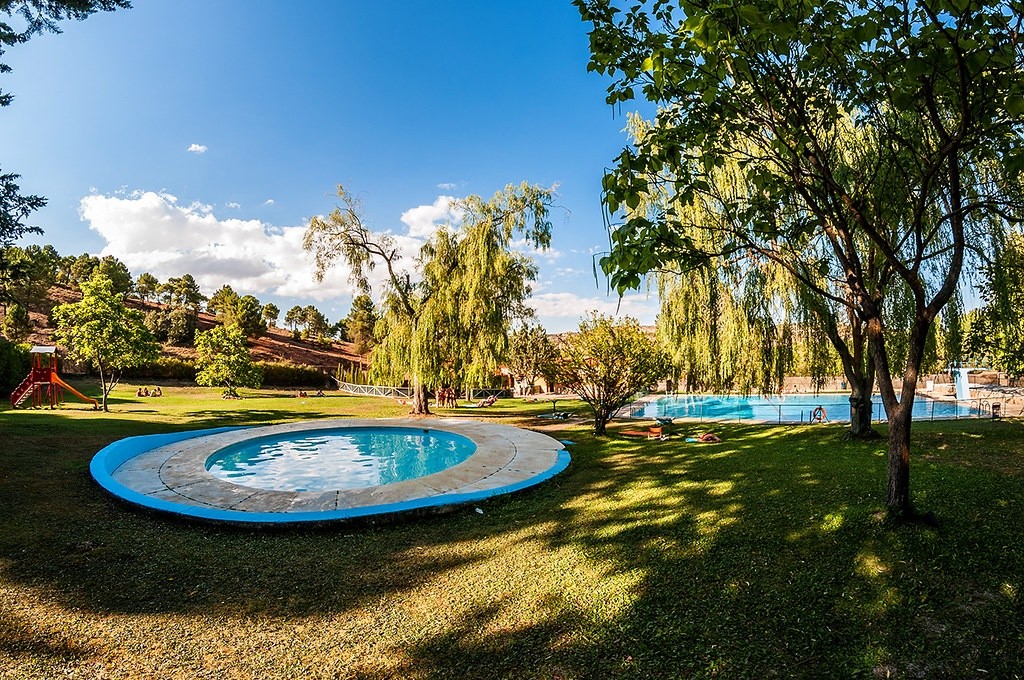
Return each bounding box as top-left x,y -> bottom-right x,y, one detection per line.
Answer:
813,407 -> 826,420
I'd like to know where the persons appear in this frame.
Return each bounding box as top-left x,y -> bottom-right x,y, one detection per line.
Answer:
143,387 -> 149,397
137,388 -> 144,397
298,389 -> 309,398
433,384 -> 497,409
150,386 -> 163,397
317,389 -> 326,398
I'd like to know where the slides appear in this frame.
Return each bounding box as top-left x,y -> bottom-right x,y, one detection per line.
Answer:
51,371 -> 99,409
